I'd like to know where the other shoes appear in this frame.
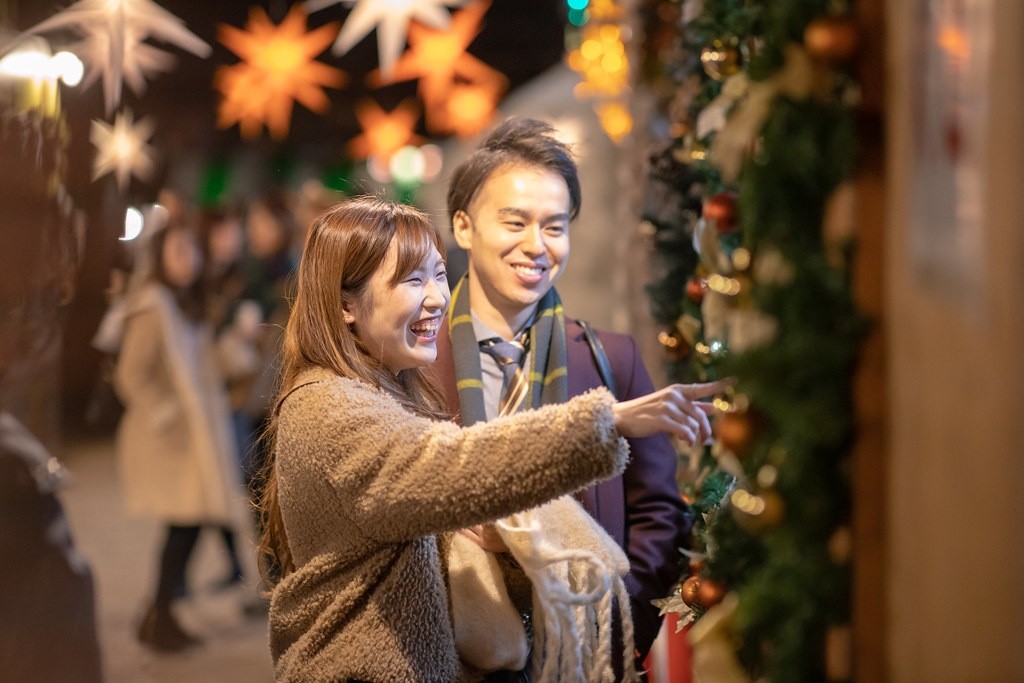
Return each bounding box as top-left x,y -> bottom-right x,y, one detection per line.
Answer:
137,611 -> 203,652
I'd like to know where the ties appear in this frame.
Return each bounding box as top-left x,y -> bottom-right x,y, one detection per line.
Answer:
478,327 -> 530,417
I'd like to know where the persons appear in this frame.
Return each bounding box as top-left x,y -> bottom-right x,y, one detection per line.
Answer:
419,115 -> 693,683
90,183 -> 331,650
258,200 -> 738,683
